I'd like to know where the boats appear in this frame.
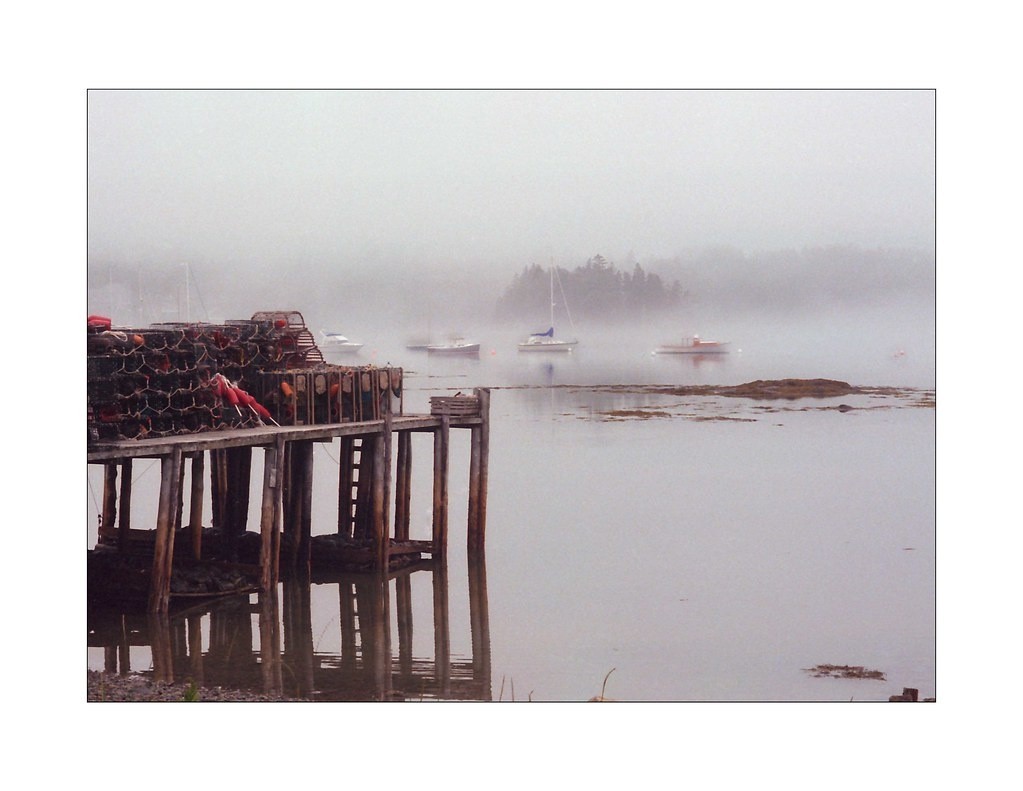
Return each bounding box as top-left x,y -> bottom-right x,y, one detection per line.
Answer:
426,340 -> 480,355
655,334 -> 731,355
406,343 -> 445,351
315,328 -> 364,353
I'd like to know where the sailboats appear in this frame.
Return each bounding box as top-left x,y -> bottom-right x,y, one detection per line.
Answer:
515,255 -> 579,353
